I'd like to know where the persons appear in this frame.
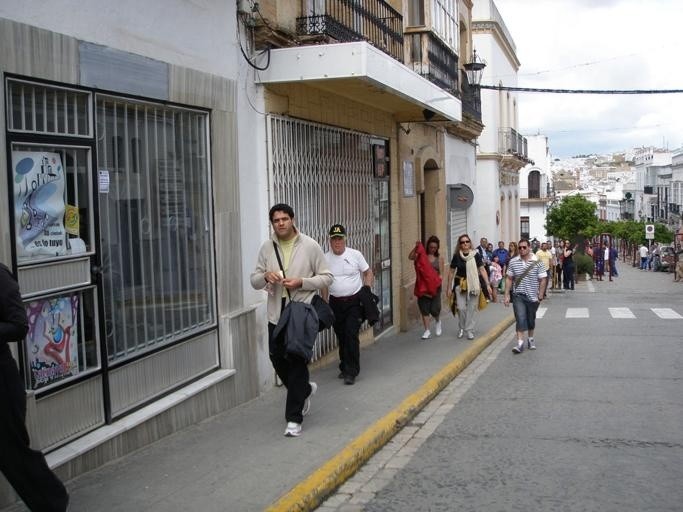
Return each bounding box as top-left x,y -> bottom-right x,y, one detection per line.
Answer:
408,235 -> 445,340
474,238 -> 683,298
320,224 -> 373,385
0,259 -> 70,511
446,234 -> 492,340
249,203 -> 334,438
503,238 -> 548,354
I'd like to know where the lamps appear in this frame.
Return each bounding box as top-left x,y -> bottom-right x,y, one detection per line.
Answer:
463,47 -> 488,105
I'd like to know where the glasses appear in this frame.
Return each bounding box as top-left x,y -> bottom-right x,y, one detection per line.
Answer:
518,245 -> 527,250
459,241 -> 470,244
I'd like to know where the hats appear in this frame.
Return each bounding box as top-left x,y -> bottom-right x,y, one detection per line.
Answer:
328,223 -> 348,239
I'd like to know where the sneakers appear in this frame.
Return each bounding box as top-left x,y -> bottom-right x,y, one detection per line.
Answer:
467,330 -> 474,340
299,381 -> 317,416
283,419 -> 303,437
434,319 -> 442,336
338,371 -> 346,379
457,328 -> 464,338
343,373 -> 355,384
527,338 -> 537,350
421,330 -> 432,339
511,341 -> 525,353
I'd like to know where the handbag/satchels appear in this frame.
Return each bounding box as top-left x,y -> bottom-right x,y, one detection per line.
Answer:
509,290 -> 514,303
307,294 -> 337,333
